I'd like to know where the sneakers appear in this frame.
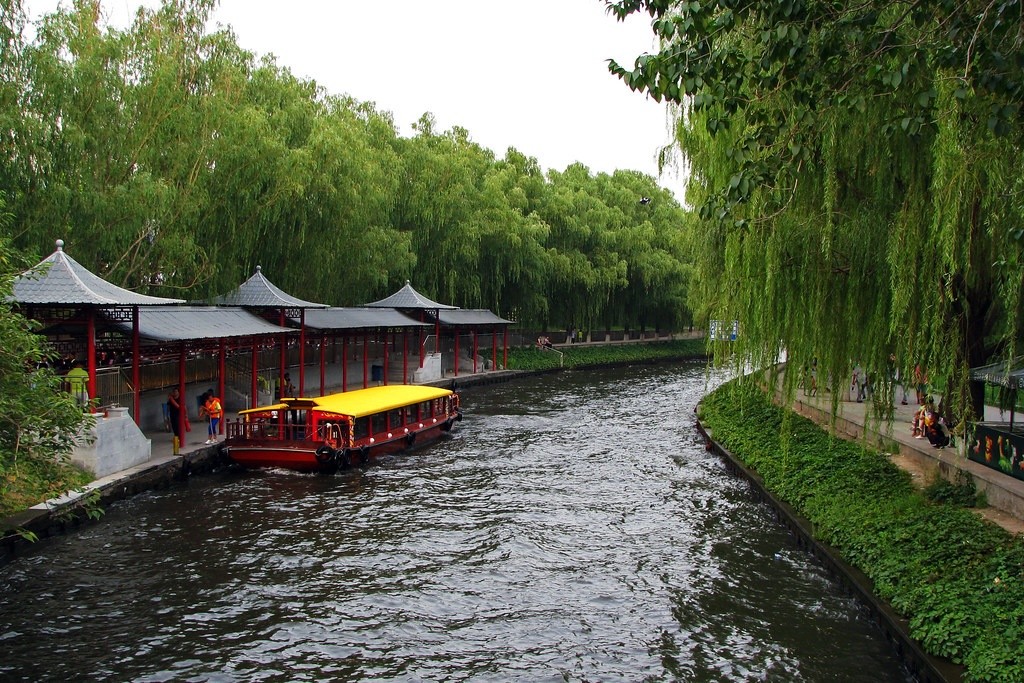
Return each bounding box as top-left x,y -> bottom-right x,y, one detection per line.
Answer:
211,439 -> 217,443
204,440 -> 214,444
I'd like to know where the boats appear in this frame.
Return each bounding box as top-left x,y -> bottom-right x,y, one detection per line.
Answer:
219,381 -> 462,473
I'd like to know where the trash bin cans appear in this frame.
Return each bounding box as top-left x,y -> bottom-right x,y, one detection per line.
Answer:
371,365 -> 384,381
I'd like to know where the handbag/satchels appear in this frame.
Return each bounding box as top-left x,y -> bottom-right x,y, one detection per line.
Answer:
186,418 -> 191,432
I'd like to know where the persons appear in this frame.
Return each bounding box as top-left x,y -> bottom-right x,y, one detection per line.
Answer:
537,335 -> 552,350
571,329 -> 583,344
166,387 -> 188,447
809,356 -> 949,450
199,388 -> 222,444
276,371 -> 295,398
64,360 -> 89,407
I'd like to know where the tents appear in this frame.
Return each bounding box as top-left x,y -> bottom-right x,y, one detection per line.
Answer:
966,354 -> 1024,435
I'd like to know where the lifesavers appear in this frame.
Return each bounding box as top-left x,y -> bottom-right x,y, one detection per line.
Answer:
344,448 -> 351,466
315,445 -> 334,464
446,418 -> 453,430
408,431 -> 416,448
360,444 -> 369,463
335,450 -> 346,470
458,413 -> 462,421
